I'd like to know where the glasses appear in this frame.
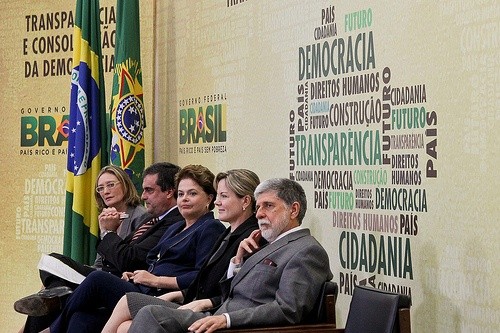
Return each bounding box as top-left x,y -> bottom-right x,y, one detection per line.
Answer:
96,181 -> 120,193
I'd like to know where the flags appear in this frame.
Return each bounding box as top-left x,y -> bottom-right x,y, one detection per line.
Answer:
107,0 -> 146,210
63,0 -> 107,266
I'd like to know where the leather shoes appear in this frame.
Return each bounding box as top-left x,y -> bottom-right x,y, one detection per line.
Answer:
14,286 -> 73,316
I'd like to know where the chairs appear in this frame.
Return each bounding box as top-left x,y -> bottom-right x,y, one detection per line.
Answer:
216,282 -> 411,333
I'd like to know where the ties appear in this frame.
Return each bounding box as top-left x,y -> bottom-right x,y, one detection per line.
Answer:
130,218 -> 159,244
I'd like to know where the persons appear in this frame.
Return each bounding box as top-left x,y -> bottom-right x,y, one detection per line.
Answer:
127,177 -> 334,333
13,161 -> 270,333
